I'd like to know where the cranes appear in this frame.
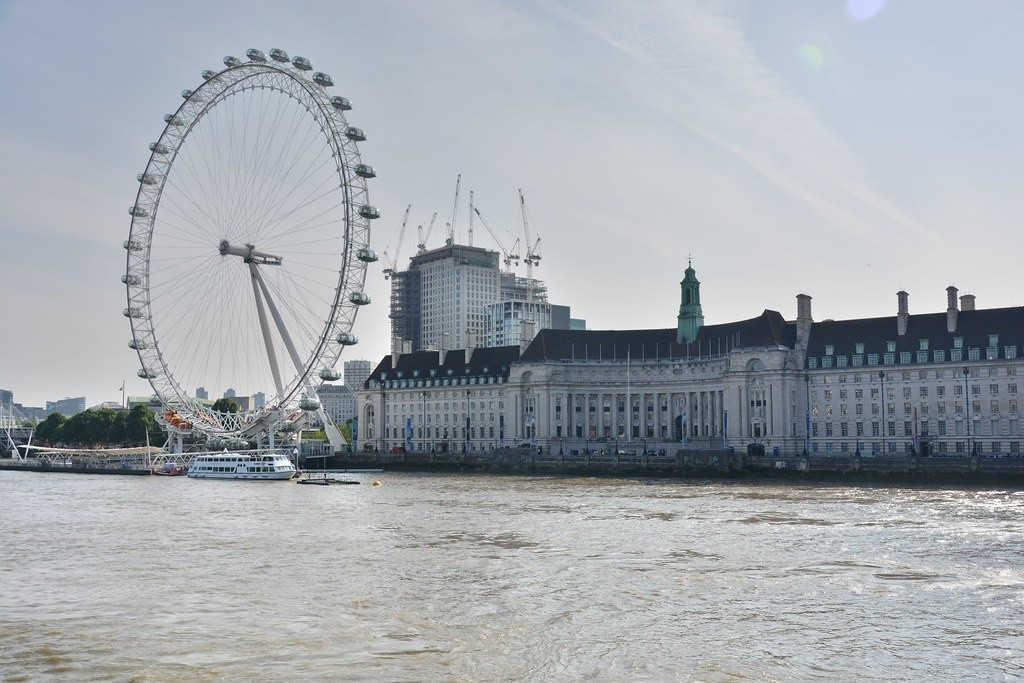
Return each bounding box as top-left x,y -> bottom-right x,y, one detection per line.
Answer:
416,174 -> 520,273
382,202 -> 413,354
517,187 -> 542,301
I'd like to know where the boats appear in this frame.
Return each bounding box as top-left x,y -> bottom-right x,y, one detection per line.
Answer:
153,463 -> 188,475
187,448 -> 297,480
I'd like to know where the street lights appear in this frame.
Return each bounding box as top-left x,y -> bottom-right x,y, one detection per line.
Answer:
879,371 -> 886,456
466,389 -> 470,454
804,374 -> 809,456
423,391 -> 426,451
963,366 -> 970,457
383,393 -> 386,453
